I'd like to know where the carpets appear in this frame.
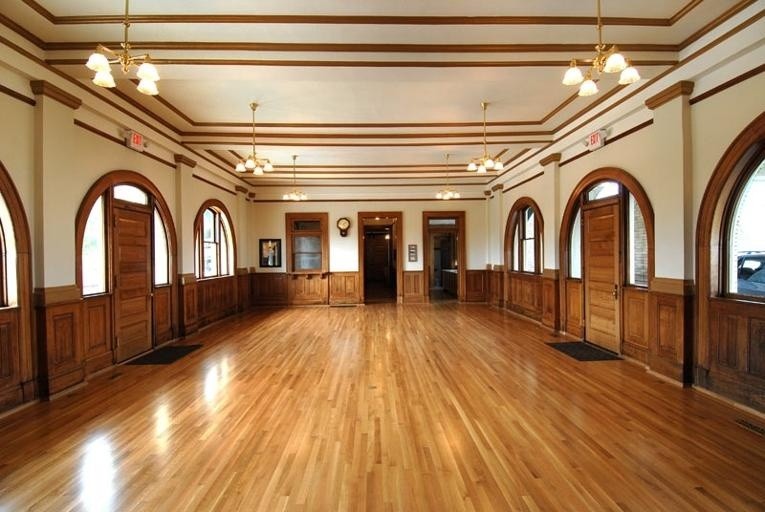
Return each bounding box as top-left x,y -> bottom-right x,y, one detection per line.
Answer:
545,341 -> 624,361
124,345 -> 202,365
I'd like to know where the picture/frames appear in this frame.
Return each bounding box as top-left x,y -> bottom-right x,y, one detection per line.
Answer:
259,239 -> 281,267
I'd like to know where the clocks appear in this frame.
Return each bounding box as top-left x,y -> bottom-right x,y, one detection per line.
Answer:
337,217 -> 350,237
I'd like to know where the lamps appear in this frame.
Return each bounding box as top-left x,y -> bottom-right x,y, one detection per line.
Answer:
466,102 -> 504,175
235,103 -> 273,176
436,154 -> 460,200
283,155 -> 307,201
85,0 -> 160,96
562,0 -> 640,96
